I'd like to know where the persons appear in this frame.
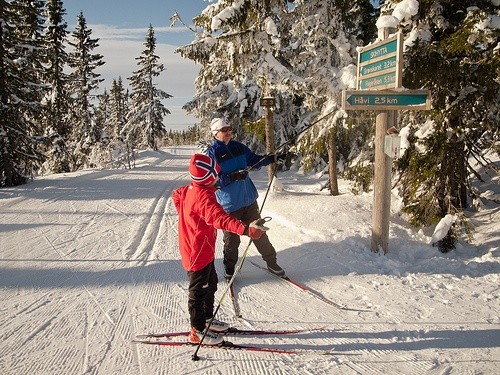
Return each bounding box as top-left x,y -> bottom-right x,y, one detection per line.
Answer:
172,154 -> 270,345
207,118 -> 287,279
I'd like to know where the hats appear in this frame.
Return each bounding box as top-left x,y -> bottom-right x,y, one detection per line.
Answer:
210,118 -> 231,137
190,154 -> 221,186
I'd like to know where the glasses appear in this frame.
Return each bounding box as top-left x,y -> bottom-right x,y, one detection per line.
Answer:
214,127 -> 232,132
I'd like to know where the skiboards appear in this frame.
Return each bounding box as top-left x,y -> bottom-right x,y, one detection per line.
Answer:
225,258 -> 346,319
130,324 -> 336,355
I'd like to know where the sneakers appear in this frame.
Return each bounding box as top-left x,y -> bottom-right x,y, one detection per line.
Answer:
206,318 -> 229,332
267,262 -> 283,273
189,328 -> 224,345
225,267 -> 234,277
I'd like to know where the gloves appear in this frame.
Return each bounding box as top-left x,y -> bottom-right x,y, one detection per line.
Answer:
231,168 -> 248,182
266,154 -> 287,164
247,219 -> 269,240
